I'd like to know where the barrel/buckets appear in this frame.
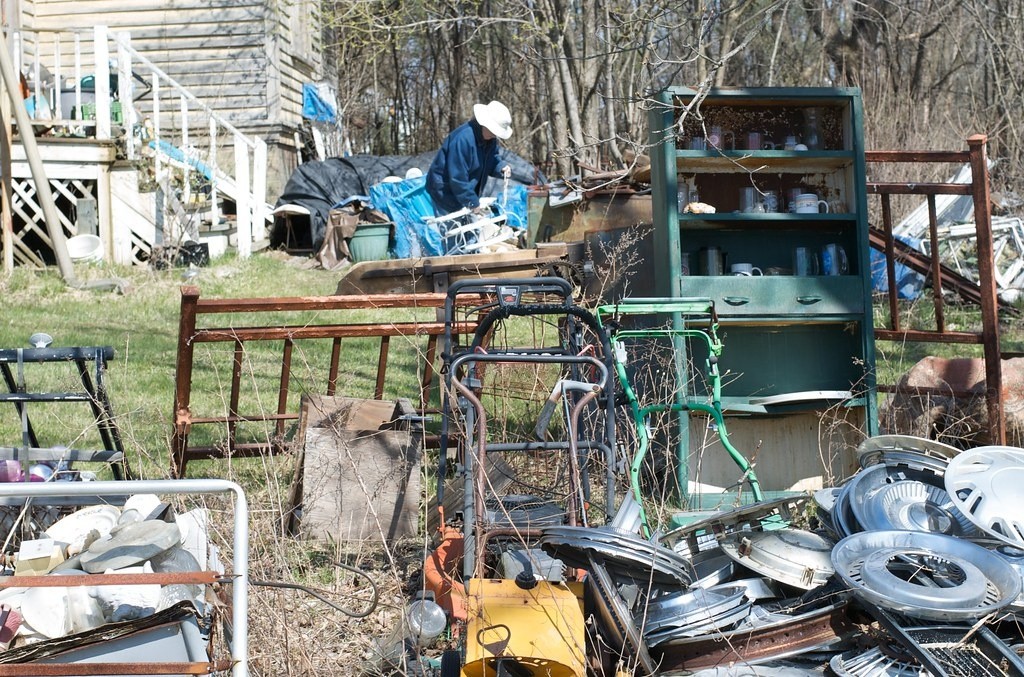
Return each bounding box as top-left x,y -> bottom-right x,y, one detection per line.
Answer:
349,224 -> 391,264
66,234 -> 104,274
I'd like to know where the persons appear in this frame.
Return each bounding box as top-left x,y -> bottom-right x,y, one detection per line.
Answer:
425,101 -> 513,256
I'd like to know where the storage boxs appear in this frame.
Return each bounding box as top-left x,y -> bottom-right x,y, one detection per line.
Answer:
155,243 -> 211,272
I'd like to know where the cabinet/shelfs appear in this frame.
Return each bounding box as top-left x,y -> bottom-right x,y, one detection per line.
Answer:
650,87 -> 880,509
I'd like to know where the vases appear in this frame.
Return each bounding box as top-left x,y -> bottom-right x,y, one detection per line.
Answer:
347,223 -> 390,262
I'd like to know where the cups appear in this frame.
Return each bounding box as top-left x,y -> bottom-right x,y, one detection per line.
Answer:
796,194 -> 829,214
744,132 -> 774,150
761,191 -> 778,213
785,188 -> 806,214
677,182 -> 688,214
791,247 -> 819,276
773,136 -> 801,151
699,246 -> 723,275
731,263 -> 763,277
704,126 -> 736,151
737,186 -> 756,213
819,242 -> 846,276
686,137 -> 704,150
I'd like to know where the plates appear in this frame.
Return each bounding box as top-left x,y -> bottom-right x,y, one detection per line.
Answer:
382,177 -> 402,182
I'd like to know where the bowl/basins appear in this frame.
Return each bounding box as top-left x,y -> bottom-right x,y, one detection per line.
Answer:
406,168 -> 422,178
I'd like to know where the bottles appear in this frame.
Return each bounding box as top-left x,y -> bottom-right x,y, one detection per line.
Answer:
410,233 -> 422,259
682,253 -> 691,276
803,109 -> 825,150
383,600 -> 446,664
777,190 -> 787,213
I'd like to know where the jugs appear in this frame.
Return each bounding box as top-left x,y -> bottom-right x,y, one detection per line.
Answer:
752,202 -> 770,213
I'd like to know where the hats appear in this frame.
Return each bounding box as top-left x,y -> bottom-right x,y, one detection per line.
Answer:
473,98 -> 513,141
